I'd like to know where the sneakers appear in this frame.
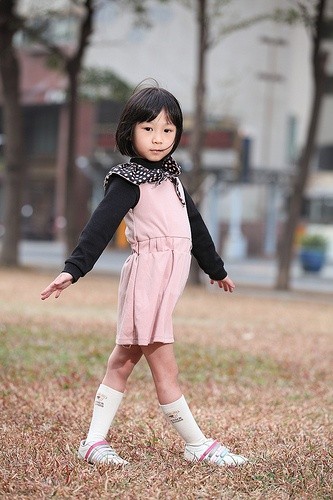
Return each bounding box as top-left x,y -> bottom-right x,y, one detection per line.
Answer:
76,438 -> 129,467
182,437 -> 250,469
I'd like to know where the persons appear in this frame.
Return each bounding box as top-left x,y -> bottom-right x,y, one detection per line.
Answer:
41,77 -> 252,470
